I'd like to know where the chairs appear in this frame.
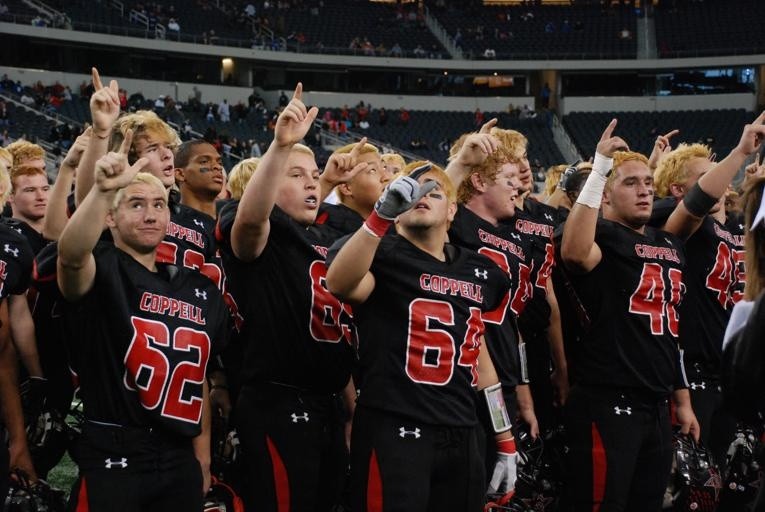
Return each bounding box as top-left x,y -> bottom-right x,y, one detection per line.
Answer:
1,85 -> 757,169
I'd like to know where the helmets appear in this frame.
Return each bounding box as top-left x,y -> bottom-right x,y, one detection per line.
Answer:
25,406 -> 66,476
64,422 -> 90,471
662,430 -> 723,512
506,471 -> 563,510
721,436 -> 764,511
4,483 -> 70,512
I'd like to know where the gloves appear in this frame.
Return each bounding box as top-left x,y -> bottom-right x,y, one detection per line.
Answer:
484,435 -> 518,507
557,159 -> 582,191
373,164 -> 436,221
